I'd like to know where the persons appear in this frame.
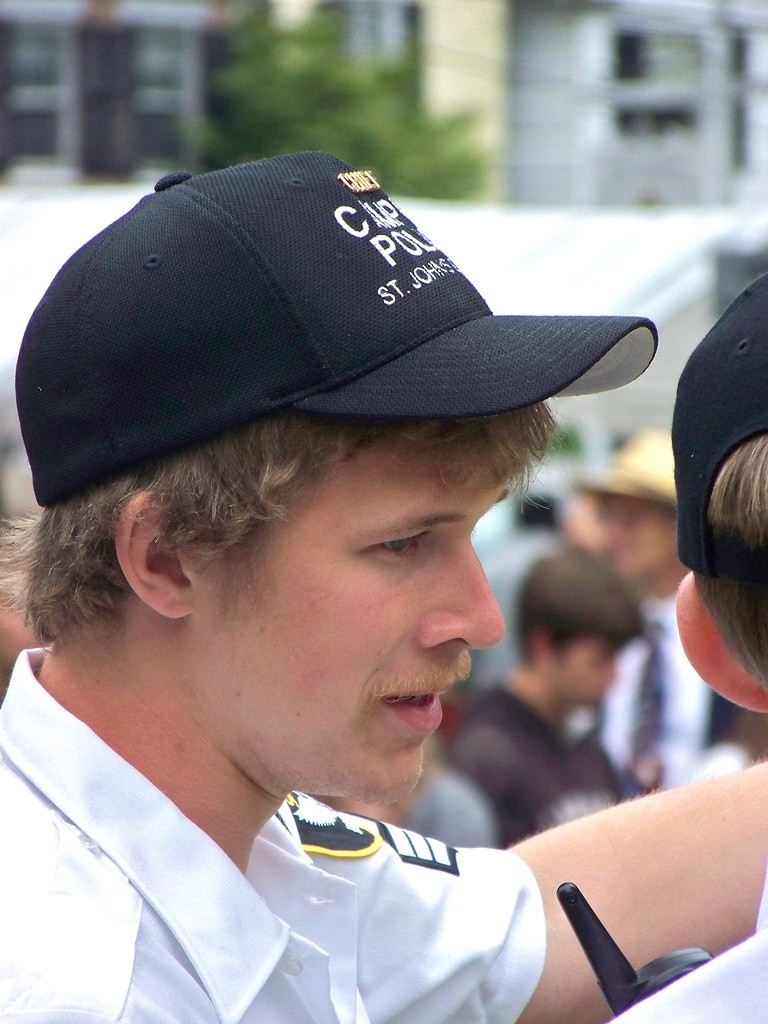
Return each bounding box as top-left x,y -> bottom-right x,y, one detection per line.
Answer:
202,2 -> 231,165
0,154 -> 768,1024
311,431 -> 768,849
608,274 -> 768,1024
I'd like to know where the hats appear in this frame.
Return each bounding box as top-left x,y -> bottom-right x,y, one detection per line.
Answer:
669,274 -> 766,587
21,145 -> 660,508
574,425 -> 680,512
